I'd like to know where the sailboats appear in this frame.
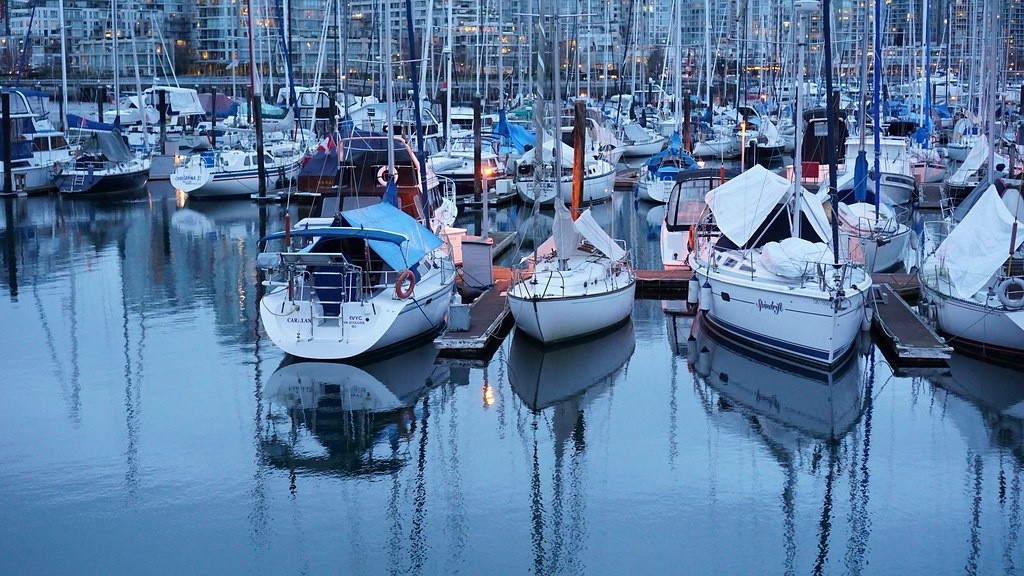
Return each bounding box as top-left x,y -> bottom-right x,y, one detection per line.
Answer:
913,0 -> 1023,355
504,0 -> 637,345
255,1 -> 457,363
0,0 -> 1024,273
693,0 -> 875,381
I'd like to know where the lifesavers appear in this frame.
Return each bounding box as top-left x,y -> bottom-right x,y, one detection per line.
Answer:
52,160 -> 62,175
688,225 -> 695,250
376,164 -> 399,186
395,270 -> 415,299
997,277 -> 1024,308
